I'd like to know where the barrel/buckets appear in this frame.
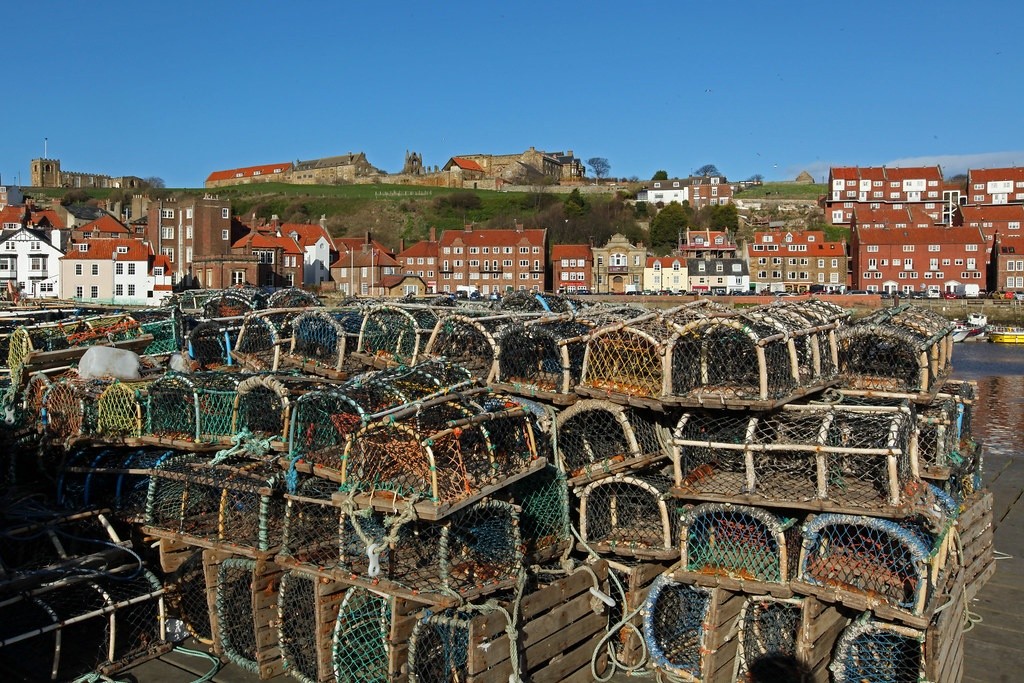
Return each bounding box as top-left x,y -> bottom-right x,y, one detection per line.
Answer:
78,347 -> 141,380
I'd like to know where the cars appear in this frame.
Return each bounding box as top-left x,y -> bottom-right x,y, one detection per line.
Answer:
404,286 -> 1024,300
